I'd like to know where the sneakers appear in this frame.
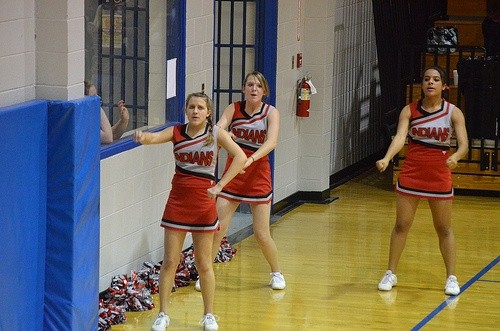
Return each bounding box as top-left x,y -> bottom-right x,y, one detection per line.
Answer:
377,270 -> 398,291
198,313 -> 220,331
151,312 -> 170,331
195,276 -> 201,291
445,275 -> 460,295
268,272 -> 286,290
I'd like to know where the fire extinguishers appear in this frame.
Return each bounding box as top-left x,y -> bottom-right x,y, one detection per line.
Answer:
294,76 -> 312,117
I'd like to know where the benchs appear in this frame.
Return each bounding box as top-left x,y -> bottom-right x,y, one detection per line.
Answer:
393,0 -> 500,190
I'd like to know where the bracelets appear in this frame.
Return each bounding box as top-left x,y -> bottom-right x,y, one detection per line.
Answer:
249,155 -> 255,162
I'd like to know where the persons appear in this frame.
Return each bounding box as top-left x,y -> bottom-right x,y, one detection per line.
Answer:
194,70 -> 286,291
375,65 -> 470,297
84,81 -> 130,145
135,92 -> 247,330
